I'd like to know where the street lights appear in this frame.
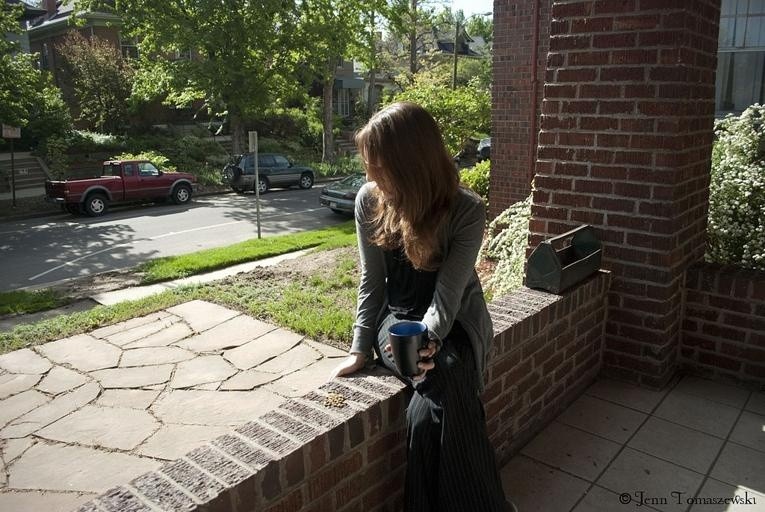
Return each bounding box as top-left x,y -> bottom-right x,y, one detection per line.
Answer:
431,11 -> 493,92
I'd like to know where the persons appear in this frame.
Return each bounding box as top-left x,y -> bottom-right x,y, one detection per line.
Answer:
331,101 -> 520,512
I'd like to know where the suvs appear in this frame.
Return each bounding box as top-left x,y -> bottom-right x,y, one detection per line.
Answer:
476,138 -> 490,163
220,153 -> 316,195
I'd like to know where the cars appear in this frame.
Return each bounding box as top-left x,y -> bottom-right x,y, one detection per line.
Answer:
319,170 -> 366,219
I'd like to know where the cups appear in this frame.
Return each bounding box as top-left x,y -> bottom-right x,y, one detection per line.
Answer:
388,321 -> 428,378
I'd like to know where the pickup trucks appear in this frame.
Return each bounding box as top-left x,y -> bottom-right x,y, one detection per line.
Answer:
44,159 -> 199,217
